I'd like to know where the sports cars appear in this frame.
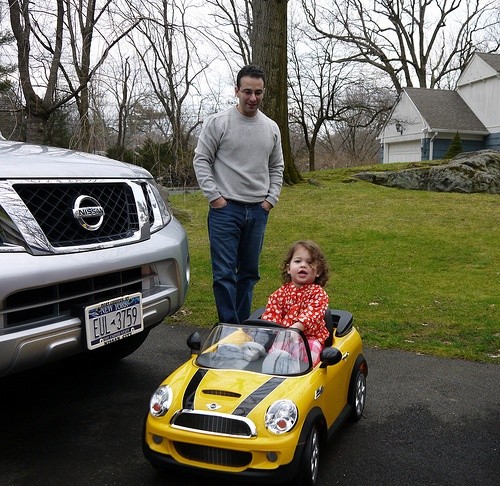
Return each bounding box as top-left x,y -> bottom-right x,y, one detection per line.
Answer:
142,305 -> 368,485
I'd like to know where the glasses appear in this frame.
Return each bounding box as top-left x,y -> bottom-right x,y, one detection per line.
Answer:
238,89 -> 265,96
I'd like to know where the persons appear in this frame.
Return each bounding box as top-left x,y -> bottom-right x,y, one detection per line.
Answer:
193,65 -> 285,326
260,241 -> 330,368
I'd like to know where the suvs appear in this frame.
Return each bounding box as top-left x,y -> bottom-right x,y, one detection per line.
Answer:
0,134 -> 191,373
